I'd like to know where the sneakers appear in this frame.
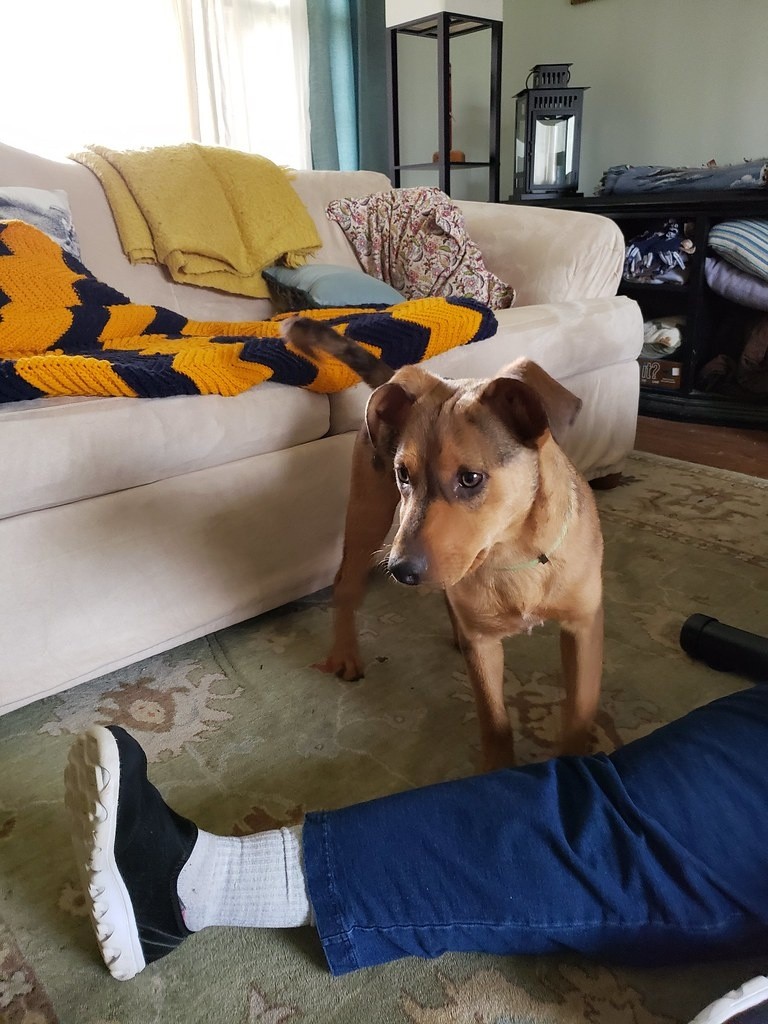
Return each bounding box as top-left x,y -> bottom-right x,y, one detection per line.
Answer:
64,724 -> 199,982
688,974 -> 768,1024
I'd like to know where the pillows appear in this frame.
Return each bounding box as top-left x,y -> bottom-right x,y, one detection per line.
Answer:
326,187 -> 516,308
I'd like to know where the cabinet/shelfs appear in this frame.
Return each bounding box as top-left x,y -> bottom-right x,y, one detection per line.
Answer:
498,188 -> 768,430
386,11 -> 505,203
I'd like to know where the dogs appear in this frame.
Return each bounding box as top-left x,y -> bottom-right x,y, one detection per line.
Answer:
279,313 -> 606,771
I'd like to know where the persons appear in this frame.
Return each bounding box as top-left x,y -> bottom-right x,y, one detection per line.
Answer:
65,684 -> 768,1024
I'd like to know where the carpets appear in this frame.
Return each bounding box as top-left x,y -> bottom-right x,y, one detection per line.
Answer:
0,449 -> 768,1024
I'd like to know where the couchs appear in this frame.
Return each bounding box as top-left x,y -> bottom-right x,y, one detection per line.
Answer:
0,142 -> 644,713
